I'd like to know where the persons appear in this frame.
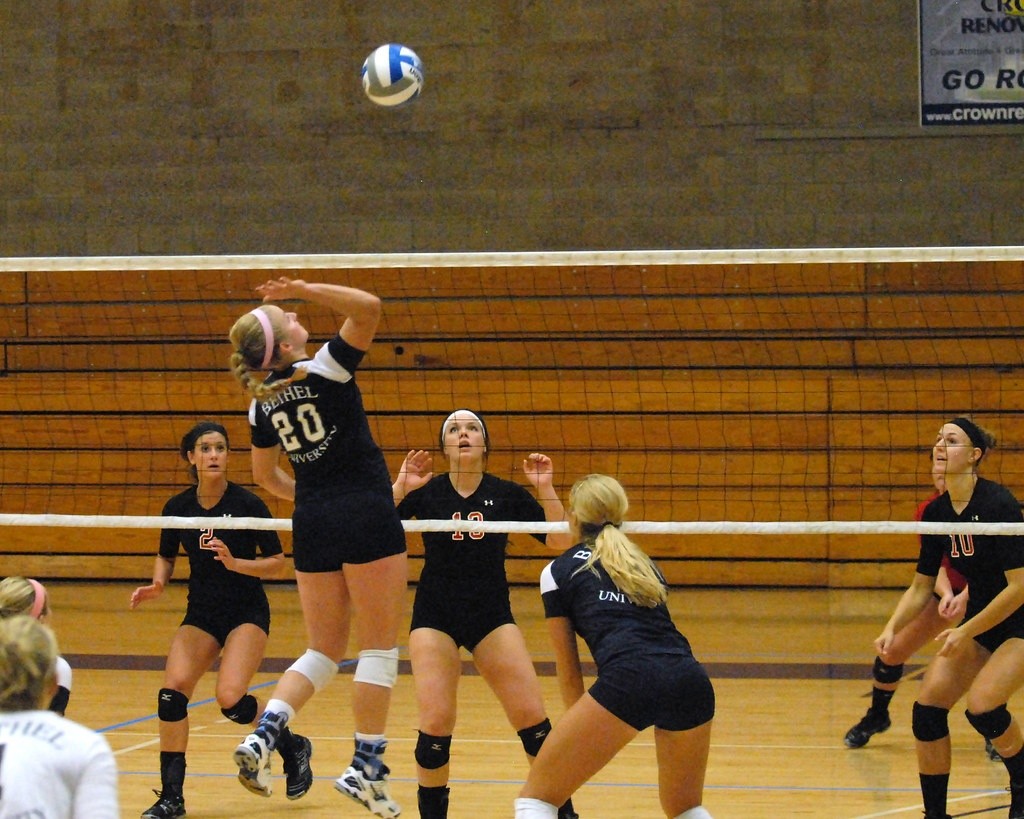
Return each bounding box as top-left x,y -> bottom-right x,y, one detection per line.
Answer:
844,451 -> 1004,762
129,418 -> 312,819
0,576 -> 73,717
227,276 -> 408,819
514,473 -> 716,819
0,614 -> 121,819
392,407 -> 580,819
871,416 -> 1024,819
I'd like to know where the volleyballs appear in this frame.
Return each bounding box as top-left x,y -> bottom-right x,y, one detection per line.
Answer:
361,41 -> 425,105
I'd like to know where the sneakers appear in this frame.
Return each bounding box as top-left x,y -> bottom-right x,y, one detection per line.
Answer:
922,810 -> 952,819
139,788 -> 186,819
1005,780 -> 1024,819
282,733 -> 314,801
333,763 -> 401,818
985,739 -> 1003,762
232,734 -> 272,797
845,706 -> 891,748
